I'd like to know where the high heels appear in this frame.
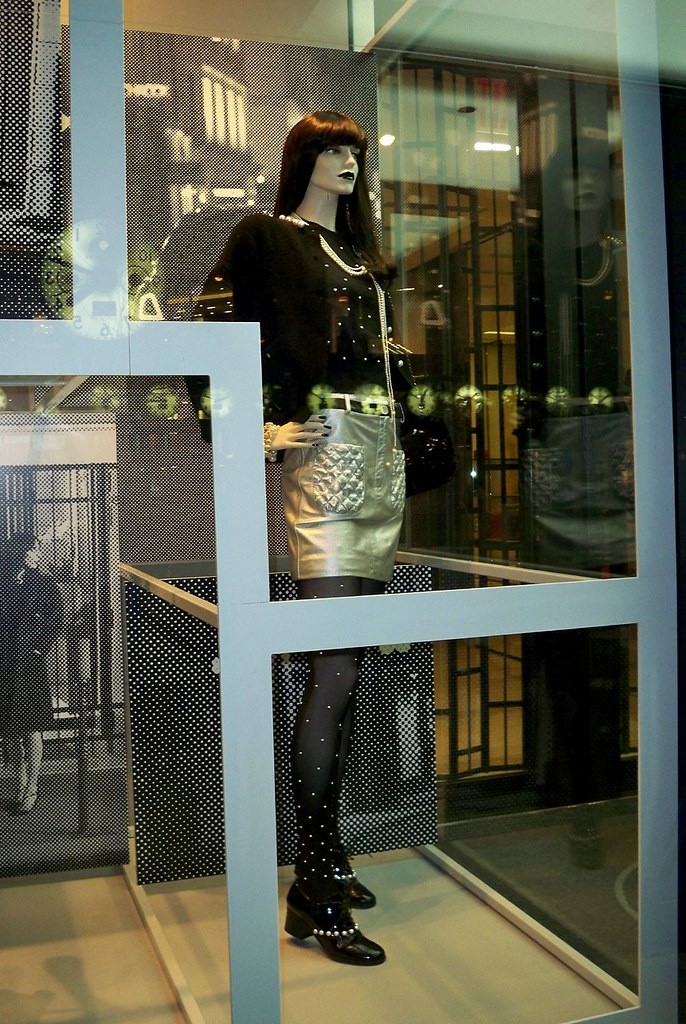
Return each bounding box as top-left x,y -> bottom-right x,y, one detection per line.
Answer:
6,787 -> 27,807
285,883 -> 385,965
334,861 -> 375,907
14,792 -> 40,815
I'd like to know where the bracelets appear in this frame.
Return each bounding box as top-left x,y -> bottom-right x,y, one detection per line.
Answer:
261,422 -> 279,463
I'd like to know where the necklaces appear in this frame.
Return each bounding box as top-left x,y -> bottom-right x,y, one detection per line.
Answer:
556,238 -> 613,393
291,211 -> 398,448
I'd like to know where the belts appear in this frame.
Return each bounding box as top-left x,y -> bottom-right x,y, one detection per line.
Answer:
317,398 -> 403,418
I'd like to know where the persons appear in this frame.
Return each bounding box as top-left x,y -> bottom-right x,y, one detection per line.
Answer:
178,110 -> 408,965
0,530 -> 61,814
513,134 -> 635,802
